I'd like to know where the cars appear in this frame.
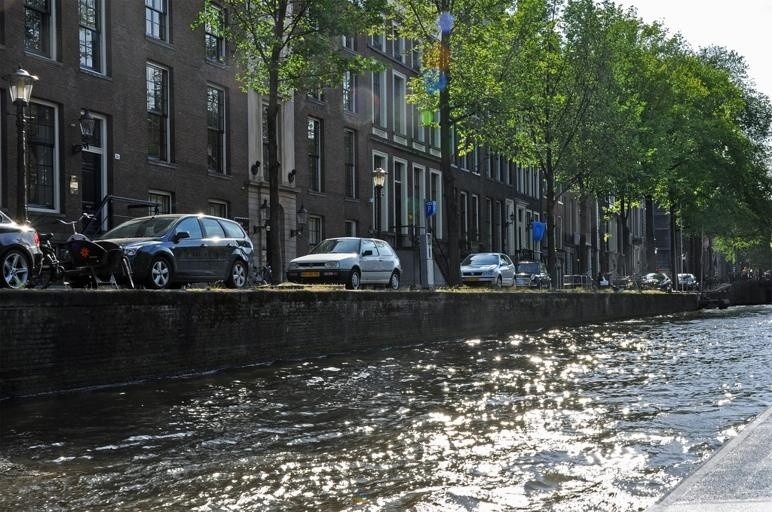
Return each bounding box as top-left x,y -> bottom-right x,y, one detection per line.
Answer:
459,252 -> 552,289
286,236 -> 401,289
639,271 -> 699,292
0,210 -> 255,289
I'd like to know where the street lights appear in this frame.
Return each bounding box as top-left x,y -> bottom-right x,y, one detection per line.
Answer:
8,65 -> 39,219
373,167 -> 388,238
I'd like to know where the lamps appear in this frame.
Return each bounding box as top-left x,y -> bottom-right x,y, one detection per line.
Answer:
253,197 -> 270,236
506,211 -> 515,226
291,201 -> 308,241
72,106 -> 95,153
